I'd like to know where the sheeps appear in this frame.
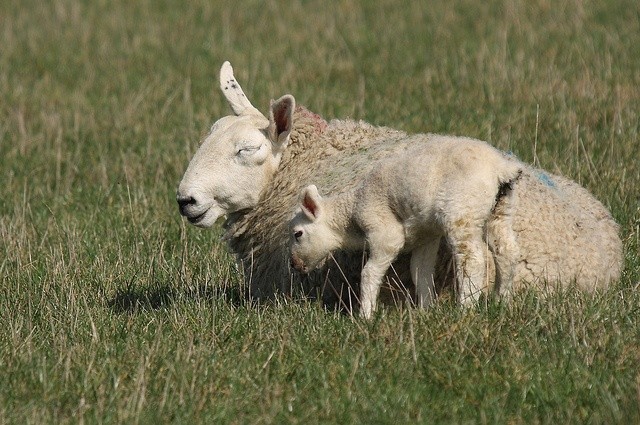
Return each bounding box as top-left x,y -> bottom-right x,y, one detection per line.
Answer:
284,132 -> 523,319
176,60 -> 625,309
